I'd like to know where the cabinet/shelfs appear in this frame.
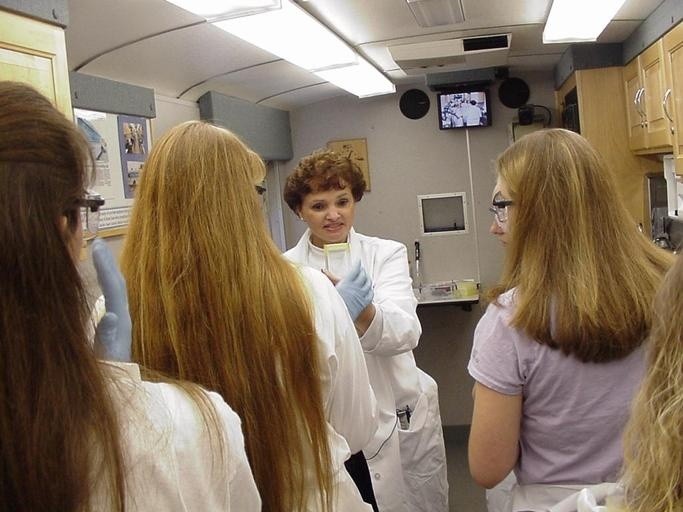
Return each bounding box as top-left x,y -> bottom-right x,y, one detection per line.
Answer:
662,21 -> 683,176
0,9 -> 74,126
554,66 -> 663,242
623,39 -> 673,156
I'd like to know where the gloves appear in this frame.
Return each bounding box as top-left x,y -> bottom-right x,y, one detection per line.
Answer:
335,258 -> 376,321
89,233 -> 135,364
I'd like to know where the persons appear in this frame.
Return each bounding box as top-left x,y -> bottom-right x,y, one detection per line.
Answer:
81,120 -> 382,512
278,149 -> 450,512
465,127 -> 677,512
543,250 -> 683,512
440,95 -> 483,127
0,79 -> 266,512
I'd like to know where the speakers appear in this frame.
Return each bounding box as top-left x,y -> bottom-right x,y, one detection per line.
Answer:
399,87 -> 431,120
498,75 -> 532,109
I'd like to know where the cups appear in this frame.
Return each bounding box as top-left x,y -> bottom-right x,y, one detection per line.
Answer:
323,242 -> 351,281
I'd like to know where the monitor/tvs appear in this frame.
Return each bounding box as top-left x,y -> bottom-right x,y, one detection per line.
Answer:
436,85 -> 493,130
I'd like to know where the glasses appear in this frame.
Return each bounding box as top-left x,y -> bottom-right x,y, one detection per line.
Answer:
69,187 -> 103,239
487,190 -> 517,225
